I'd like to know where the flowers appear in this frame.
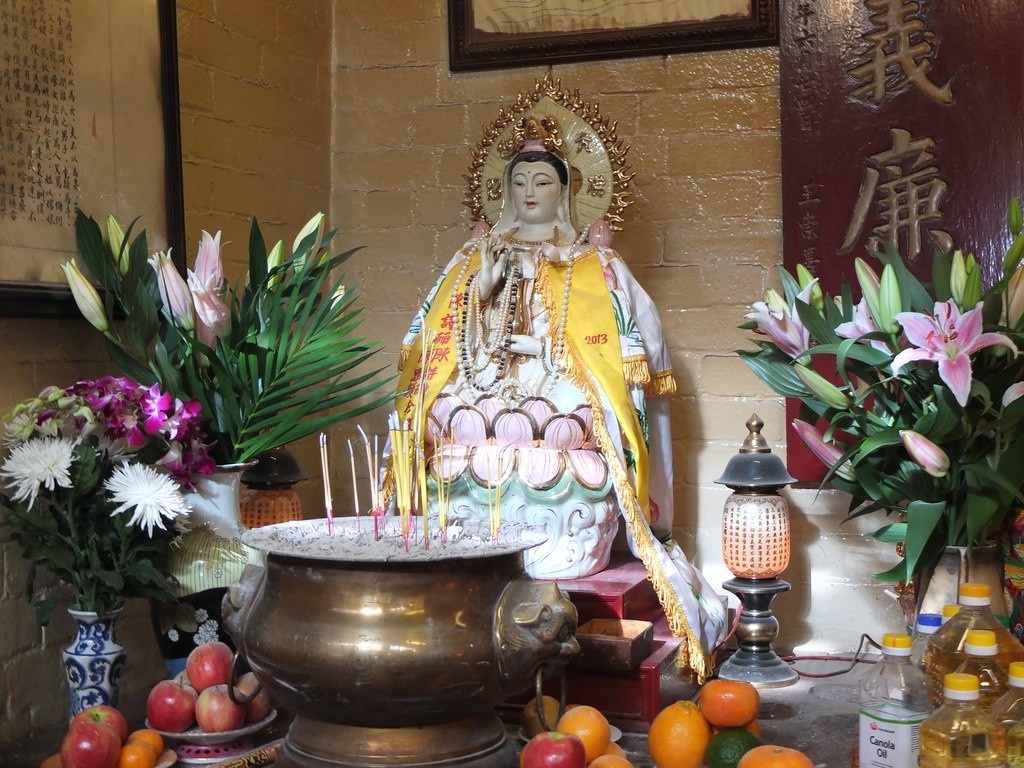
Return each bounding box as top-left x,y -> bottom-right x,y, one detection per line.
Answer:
0,365 -> 216,607
59,209 -> 381,461
736,203 -> 1023,589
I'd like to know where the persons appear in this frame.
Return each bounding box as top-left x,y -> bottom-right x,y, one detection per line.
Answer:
388,146 -> 644,423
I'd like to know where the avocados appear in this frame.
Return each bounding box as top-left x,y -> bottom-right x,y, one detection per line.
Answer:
704,726 -> 766,768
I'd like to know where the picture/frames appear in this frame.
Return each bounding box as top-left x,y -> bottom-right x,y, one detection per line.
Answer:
444,1 -> 780,73
3,1 -> 187,326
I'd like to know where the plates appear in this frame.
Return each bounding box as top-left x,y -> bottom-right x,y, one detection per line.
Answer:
142,706 -> 277,746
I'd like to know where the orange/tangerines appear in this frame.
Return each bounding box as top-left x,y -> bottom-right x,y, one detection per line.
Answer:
555,705 -> 633,768
646,679 -> 813,768
118,729 -> 164,768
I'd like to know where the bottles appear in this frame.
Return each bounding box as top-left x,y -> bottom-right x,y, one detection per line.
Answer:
924,583 -> 1024,714
858,633 -> 939,768
953,629 -> 1009,713
989,661 -> 1024,768
917,671 -> 1008,768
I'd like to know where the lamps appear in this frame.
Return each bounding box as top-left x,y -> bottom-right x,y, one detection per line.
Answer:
715,415 -> 801,685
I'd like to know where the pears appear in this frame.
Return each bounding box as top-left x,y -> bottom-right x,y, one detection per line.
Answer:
521,695 -> 561,738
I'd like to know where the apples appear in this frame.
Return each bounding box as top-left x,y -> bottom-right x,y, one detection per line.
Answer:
145,641 -> 269,733
60,705 -> 128,768
521,731 -> 585,768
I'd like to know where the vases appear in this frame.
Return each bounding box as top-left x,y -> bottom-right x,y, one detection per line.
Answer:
61,609 -> 125,727
149,462 -> 263,675
912,543 -> 999,657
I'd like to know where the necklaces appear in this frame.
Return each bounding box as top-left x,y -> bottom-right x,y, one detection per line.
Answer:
448,215 -> 592,400
